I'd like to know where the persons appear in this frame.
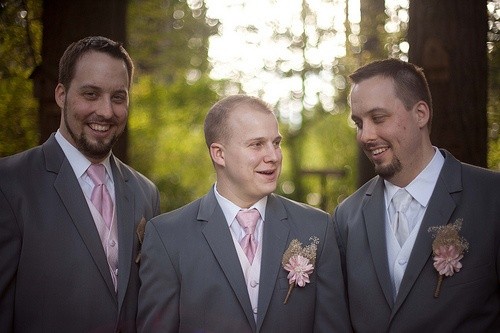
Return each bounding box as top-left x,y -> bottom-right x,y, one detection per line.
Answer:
137,96 -> 351,333
0,37 -> 163,333
337,58 -> 500,333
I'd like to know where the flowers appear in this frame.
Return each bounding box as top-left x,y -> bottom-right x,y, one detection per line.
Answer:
282,236 -> 320,305
428,219 -> 470,298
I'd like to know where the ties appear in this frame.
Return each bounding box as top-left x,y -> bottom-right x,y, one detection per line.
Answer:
235,210 -> 260,266
86,164 -> 113,230
392,190 -> 412,248
